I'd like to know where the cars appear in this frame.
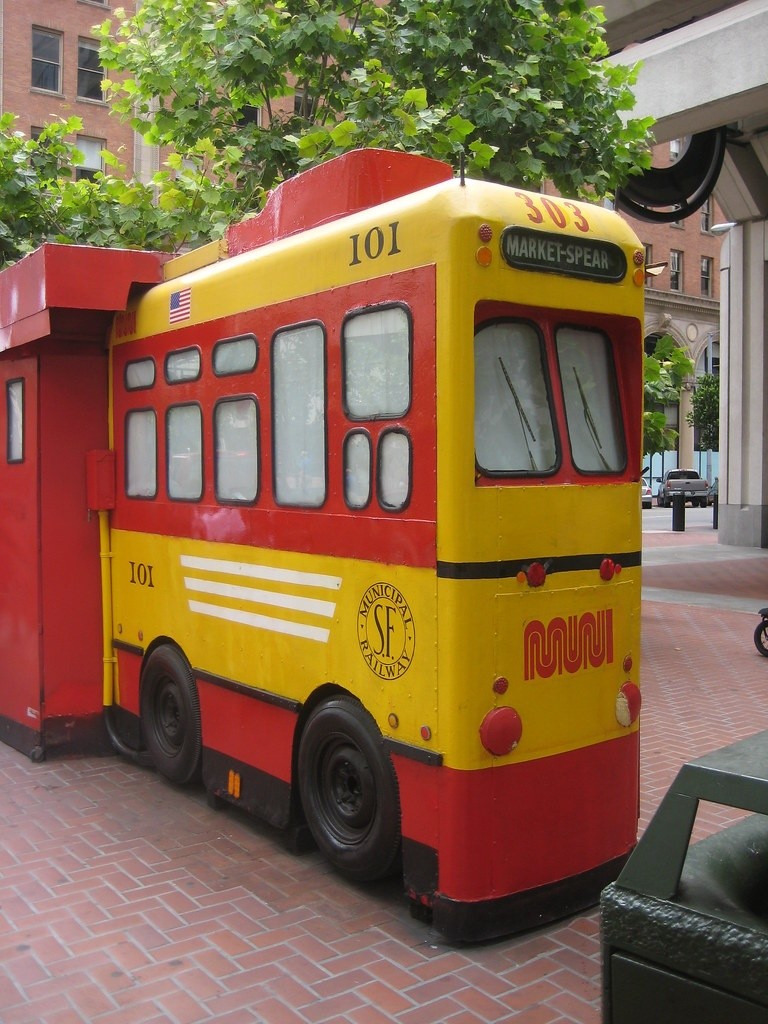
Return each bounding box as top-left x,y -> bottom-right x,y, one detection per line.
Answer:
642,477 -> 653,508
708,478 -> 718,506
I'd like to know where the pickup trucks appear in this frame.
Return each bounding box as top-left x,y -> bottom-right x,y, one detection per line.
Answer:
656,468 -> 709,508
